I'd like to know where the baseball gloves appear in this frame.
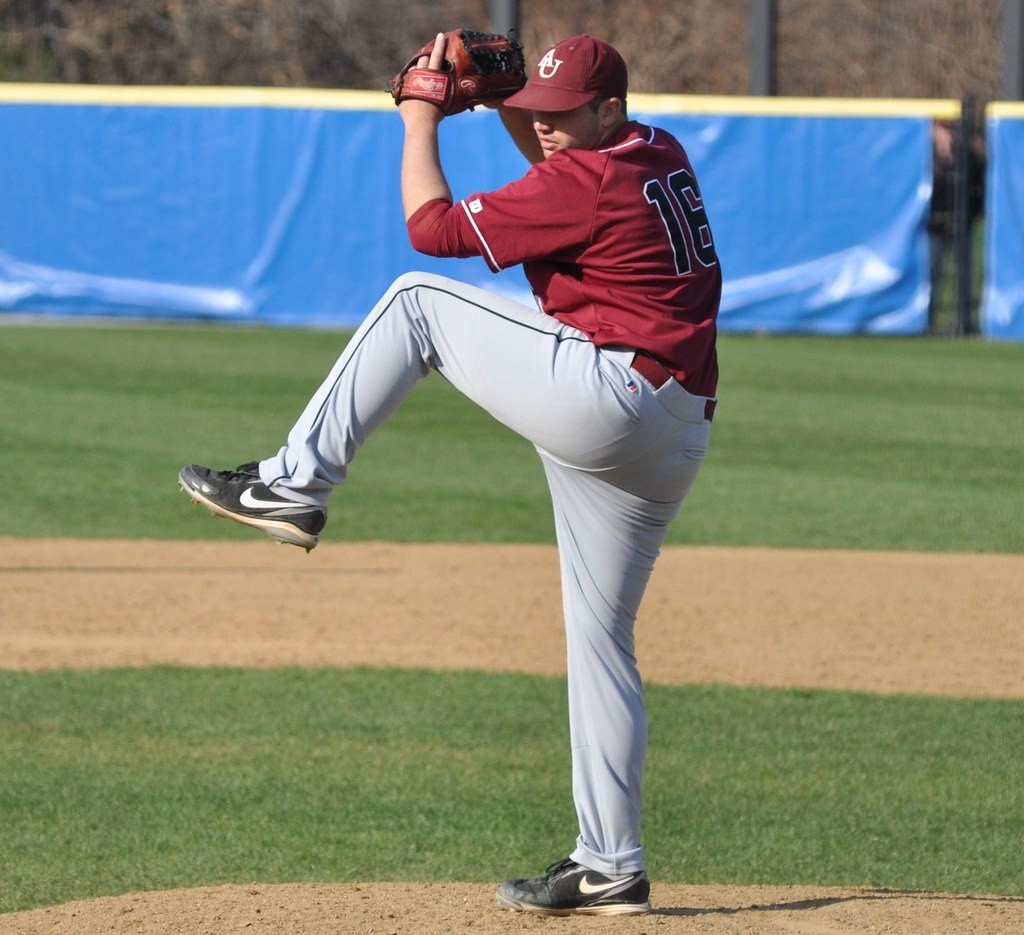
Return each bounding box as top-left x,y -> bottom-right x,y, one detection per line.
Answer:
383,25 -> 529,119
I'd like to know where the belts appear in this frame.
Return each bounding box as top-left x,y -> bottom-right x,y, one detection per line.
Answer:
631,352 -> 717,423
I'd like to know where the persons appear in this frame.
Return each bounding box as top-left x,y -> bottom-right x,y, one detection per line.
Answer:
175,30 -> 723,915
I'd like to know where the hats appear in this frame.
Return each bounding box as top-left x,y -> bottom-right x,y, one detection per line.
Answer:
503,35 -> 629,112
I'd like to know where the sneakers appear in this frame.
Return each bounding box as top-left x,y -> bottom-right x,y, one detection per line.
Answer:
177,461 -> 326,554
495,857 -> 650,916
